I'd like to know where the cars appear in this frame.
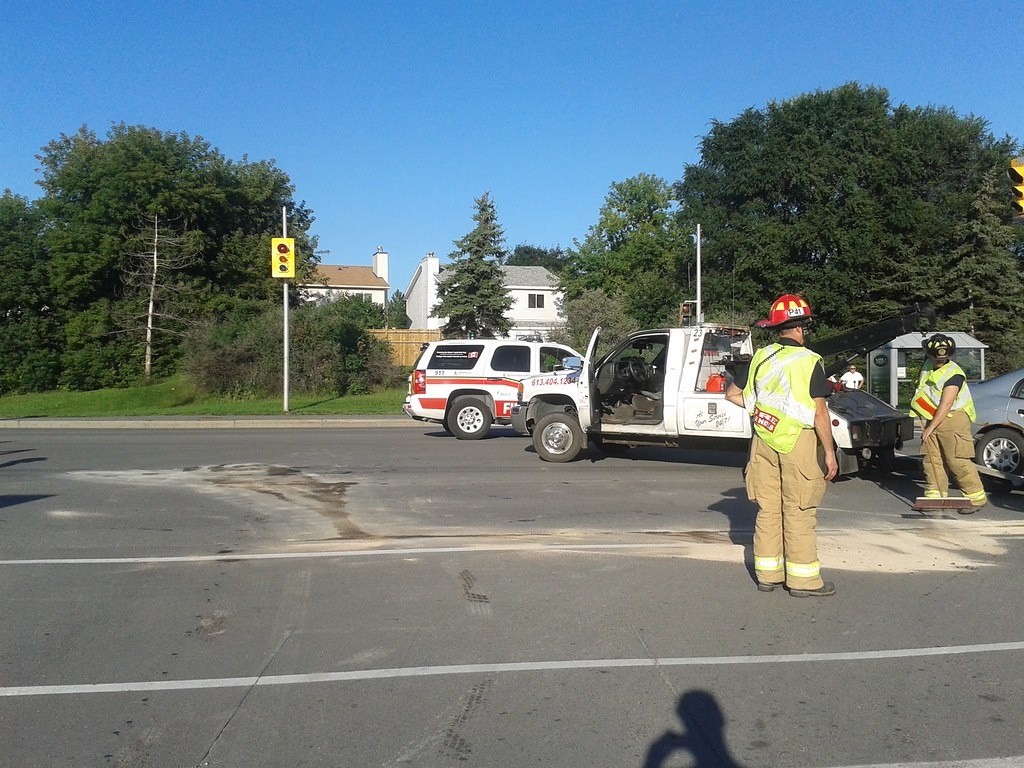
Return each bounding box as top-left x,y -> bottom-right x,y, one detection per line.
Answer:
965,367 -> 1024,494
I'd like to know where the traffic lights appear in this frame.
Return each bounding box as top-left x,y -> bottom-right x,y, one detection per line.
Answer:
678,303 -> 693,326
1006,157 -> 1024,218
271,237 -> 296,278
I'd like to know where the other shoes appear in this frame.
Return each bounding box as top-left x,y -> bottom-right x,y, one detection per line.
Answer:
959,502 -> 987,514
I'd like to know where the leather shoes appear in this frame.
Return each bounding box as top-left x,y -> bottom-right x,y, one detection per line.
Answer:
789,580 -> 836,597
758,580 -> 783,591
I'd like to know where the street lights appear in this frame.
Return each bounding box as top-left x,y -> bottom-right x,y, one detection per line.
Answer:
689,223 -> 708,324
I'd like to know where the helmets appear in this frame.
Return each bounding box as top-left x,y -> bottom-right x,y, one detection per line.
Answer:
922,334 -> 956,360
757,294 -> 818,327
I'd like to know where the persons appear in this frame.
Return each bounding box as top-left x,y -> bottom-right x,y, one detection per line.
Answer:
909,333 -> 988,513
839,365 -> 864,389
725,294 -> 838,598
827,374 -> 837,382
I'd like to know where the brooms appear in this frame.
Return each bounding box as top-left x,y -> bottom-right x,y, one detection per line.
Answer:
912,416 -> 975,509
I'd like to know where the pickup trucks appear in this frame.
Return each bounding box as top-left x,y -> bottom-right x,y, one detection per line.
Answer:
509,319 -> 916,478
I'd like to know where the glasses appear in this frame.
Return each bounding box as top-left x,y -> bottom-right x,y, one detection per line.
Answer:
850,368 -> 856,370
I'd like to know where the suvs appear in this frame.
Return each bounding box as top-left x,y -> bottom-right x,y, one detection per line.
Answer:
401,338 -> 586,440
